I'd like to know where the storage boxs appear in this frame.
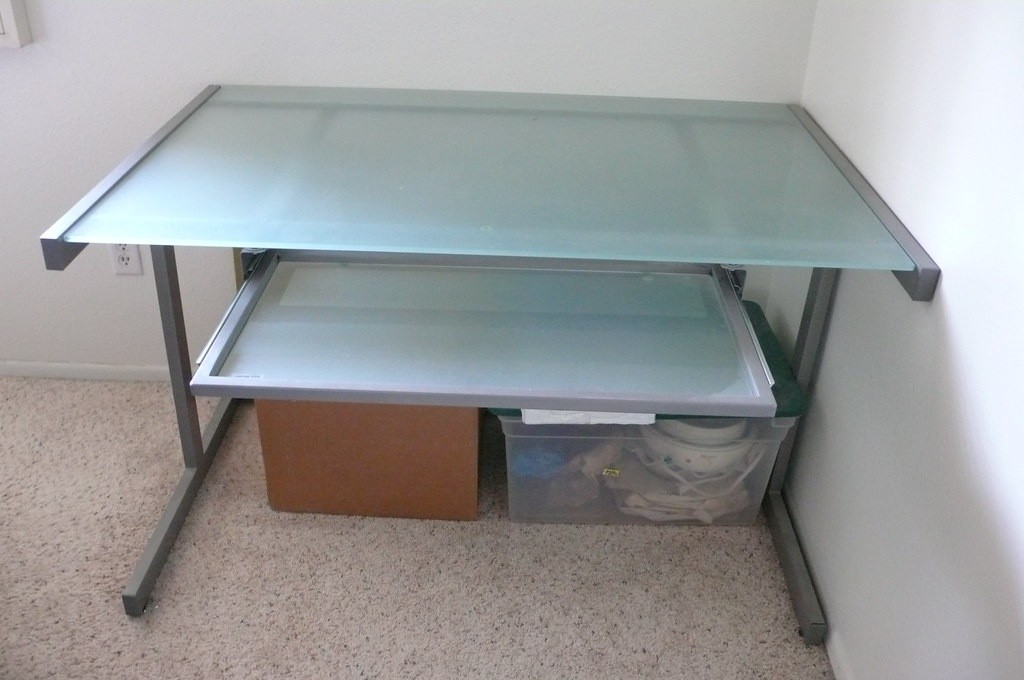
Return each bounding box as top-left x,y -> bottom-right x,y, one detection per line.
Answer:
485,300 -> 806,528
254,398 -> 479,521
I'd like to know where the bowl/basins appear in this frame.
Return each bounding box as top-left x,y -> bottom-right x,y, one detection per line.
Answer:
640,424 -> 759,472
656,418 -> 747,445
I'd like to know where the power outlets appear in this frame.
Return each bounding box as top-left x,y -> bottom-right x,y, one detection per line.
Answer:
110,244 -> 143,276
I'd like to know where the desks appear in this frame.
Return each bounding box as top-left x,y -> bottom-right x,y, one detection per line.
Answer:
37,83 -> 942,648
